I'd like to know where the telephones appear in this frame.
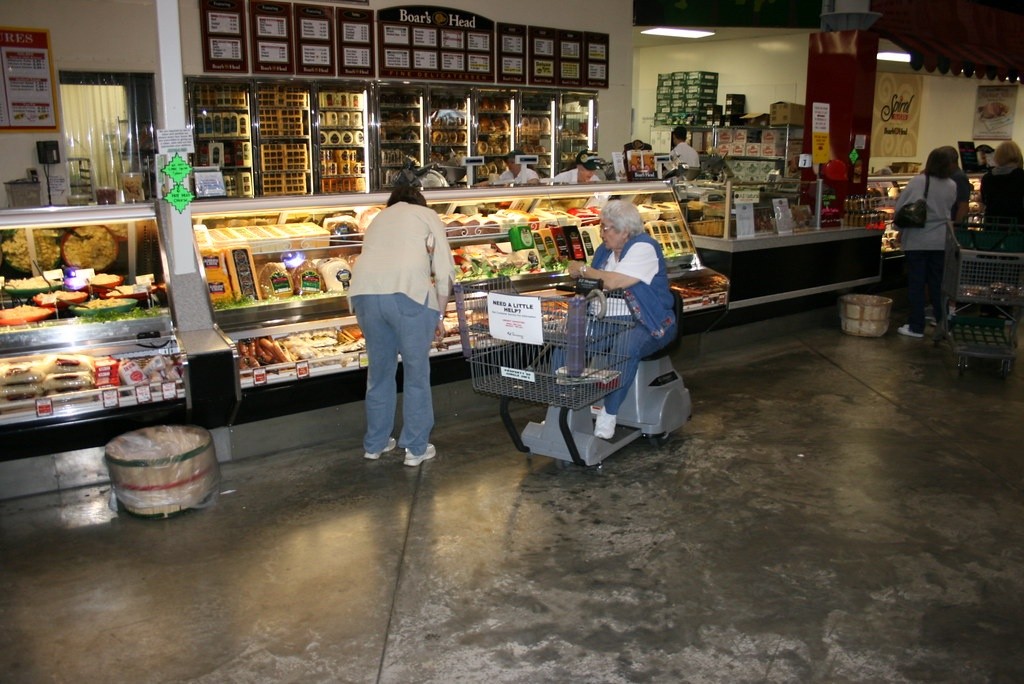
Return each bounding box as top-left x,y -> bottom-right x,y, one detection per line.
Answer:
36,140 -> 60,165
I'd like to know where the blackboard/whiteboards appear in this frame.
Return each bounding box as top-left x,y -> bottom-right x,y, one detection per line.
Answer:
958,141 -> 978,170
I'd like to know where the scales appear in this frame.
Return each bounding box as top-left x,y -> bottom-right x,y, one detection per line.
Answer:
461,156 -> 484,186
515,155 -> 539,183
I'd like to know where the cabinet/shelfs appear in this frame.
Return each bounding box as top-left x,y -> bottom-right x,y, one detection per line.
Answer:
67,157 -> 97,204
654,125 -> 804,180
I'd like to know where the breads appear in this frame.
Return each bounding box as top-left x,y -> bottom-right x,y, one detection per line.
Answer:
278,325 -> 365,361
323,207 -> 502,246
257,257 -> 357,301
379,93 -> 549,175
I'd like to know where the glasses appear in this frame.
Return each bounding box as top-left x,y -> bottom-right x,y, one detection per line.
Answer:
599,225 -> 615,232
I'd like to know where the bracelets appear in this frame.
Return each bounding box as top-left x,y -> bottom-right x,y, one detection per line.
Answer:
438,314 -> 444,321
579,265 -> 589,278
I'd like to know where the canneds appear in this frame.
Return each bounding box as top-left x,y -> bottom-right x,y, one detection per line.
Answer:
382,150 -> 402,183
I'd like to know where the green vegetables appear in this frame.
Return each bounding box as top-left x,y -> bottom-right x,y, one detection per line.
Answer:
452,256 -> 568,282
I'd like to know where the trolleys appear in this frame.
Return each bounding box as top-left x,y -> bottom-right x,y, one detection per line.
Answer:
937,220 -> 1023,381
452,267 -> 696,476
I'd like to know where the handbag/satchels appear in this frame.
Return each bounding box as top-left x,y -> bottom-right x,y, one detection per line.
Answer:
893,170 -> 930,231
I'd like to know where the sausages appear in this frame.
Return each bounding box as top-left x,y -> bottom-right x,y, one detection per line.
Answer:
237,338 -> 291,372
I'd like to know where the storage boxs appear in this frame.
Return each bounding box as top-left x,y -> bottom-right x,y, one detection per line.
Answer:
654,71 -> 805,181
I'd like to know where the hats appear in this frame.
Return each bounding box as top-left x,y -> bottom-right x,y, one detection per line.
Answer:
576,148 -> 603,171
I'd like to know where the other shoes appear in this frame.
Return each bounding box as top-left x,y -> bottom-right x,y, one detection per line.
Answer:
895,323 -> 928,338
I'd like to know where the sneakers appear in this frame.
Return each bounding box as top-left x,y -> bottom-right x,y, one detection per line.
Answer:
403,443 -> 436,466
594,406 -> 616,439
363,437 -> 396,460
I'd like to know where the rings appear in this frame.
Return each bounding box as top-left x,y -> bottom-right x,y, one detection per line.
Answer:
441,337 -> 443,339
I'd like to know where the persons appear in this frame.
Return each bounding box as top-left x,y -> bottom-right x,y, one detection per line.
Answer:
554,149 -> 604,184
895,146 -> 970,338
669,126 -> 700,181
499,149 -> 539,183
971,141 -> 1024,260
350,184 -> 456,465
540,200 -> 677,440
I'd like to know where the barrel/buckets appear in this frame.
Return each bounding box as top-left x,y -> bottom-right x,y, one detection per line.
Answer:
104,425 -> 218,517
839,294 -> 893,337
